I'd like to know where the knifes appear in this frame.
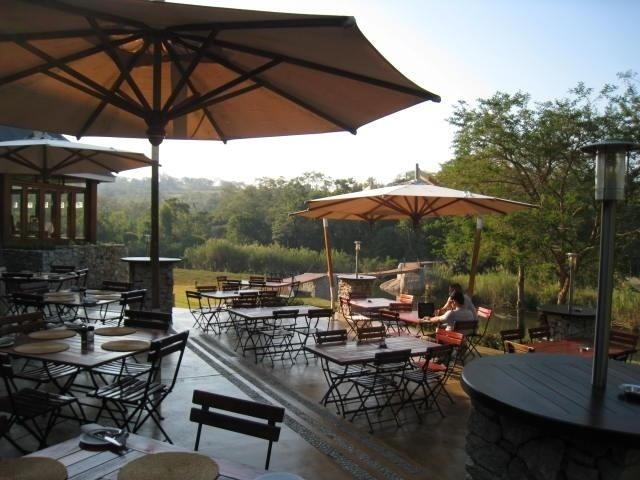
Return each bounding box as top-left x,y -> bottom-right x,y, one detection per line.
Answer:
95,433 -> 122,447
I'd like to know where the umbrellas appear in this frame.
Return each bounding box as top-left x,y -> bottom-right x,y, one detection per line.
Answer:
1,0 -> 440,311
0,134 -> 160,246
289,177 -> 434,271
306,164 -> 545,303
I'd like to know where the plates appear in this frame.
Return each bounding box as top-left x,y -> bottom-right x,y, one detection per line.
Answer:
256,472 -> 304,480
619,384 -> 640,396
0,338 -> 15,348
79,428 -> 122,445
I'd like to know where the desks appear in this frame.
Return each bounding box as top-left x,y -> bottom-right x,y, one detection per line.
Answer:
17,221 -> 54,235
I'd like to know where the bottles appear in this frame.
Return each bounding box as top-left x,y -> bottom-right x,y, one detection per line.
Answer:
80,322 -> 94,348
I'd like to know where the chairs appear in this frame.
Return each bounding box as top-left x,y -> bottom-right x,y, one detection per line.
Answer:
11,214 -> 21,234
30,215 -> 38,224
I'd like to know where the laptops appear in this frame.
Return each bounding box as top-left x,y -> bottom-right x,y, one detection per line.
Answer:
417,303 -> 436,319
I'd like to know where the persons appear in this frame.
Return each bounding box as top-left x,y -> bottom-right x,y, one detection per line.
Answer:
423,292 -> 474,364
439,283 -> 477,321
